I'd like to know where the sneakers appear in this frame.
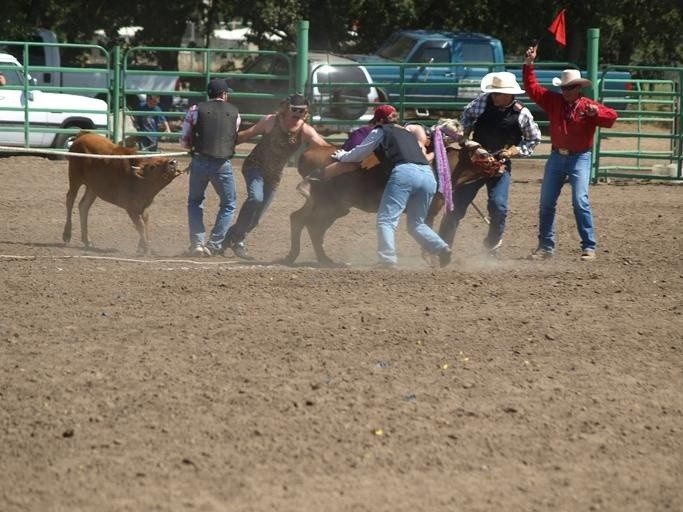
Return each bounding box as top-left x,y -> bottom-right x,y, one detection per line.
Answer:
372,262 -> 396,270
440,247 -> 452,268
185,240 -> 253,259
580,249 -> 595,260
526,248 -> 554,259
486,248 -> 509,259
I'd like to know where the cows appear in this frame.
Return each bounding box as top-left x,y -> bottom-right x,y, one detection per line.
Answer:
62,134 -> 178,255
283,130 -> 508,268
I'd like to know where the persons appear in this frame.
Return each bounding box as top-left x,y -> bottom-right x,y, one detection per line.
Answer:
438,72 -> 543,256
222,90 -> 330,257
342,116 -> 466,150
181,79 -> 242,255
134,93 -> 170,151
330,105 -> 452,268
521,46 -> 617,260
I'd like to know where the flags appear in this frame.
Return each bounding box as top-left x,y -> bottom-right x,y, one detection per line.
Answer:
549,11 -> 567,48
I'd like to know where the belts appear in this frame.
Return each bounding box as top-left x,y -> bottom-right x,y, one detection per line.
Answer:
552,146 -> 592,155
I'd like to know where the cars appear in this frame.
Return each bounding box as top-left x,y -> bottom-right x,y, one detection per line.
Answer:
0,53 -> 110,160
223,51 -> 379,137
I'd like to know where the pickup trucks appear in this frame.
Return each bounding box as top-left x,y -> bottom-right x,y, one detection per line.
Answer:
342,31 -> 633,134
0,29 -> 180,115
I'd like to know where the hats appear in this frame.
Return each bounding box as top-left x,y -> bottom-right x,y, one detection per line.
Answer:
369,105 -> 396,122
208,78 -> 228,95
553,69 -> 592,88
481,72 -> 526,94
